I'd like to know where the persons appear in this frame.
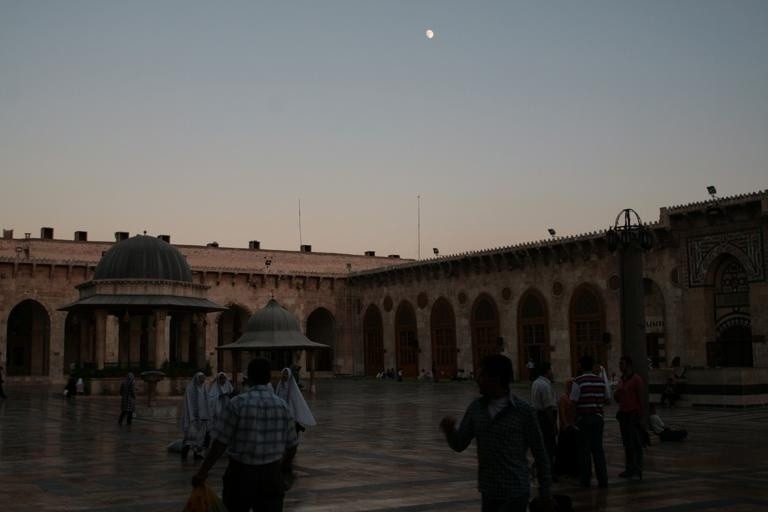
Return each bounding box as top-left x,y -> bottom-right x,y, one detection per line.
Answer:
648,403 -> 688,441
0,352 -> 8,400
118,373 -> 136,432
62,360 -> 90,401
181,357 -> 318,512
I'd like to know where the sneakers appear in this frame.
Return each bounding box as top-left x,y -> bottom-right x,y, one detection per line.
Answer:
619,466 -> 643,479
